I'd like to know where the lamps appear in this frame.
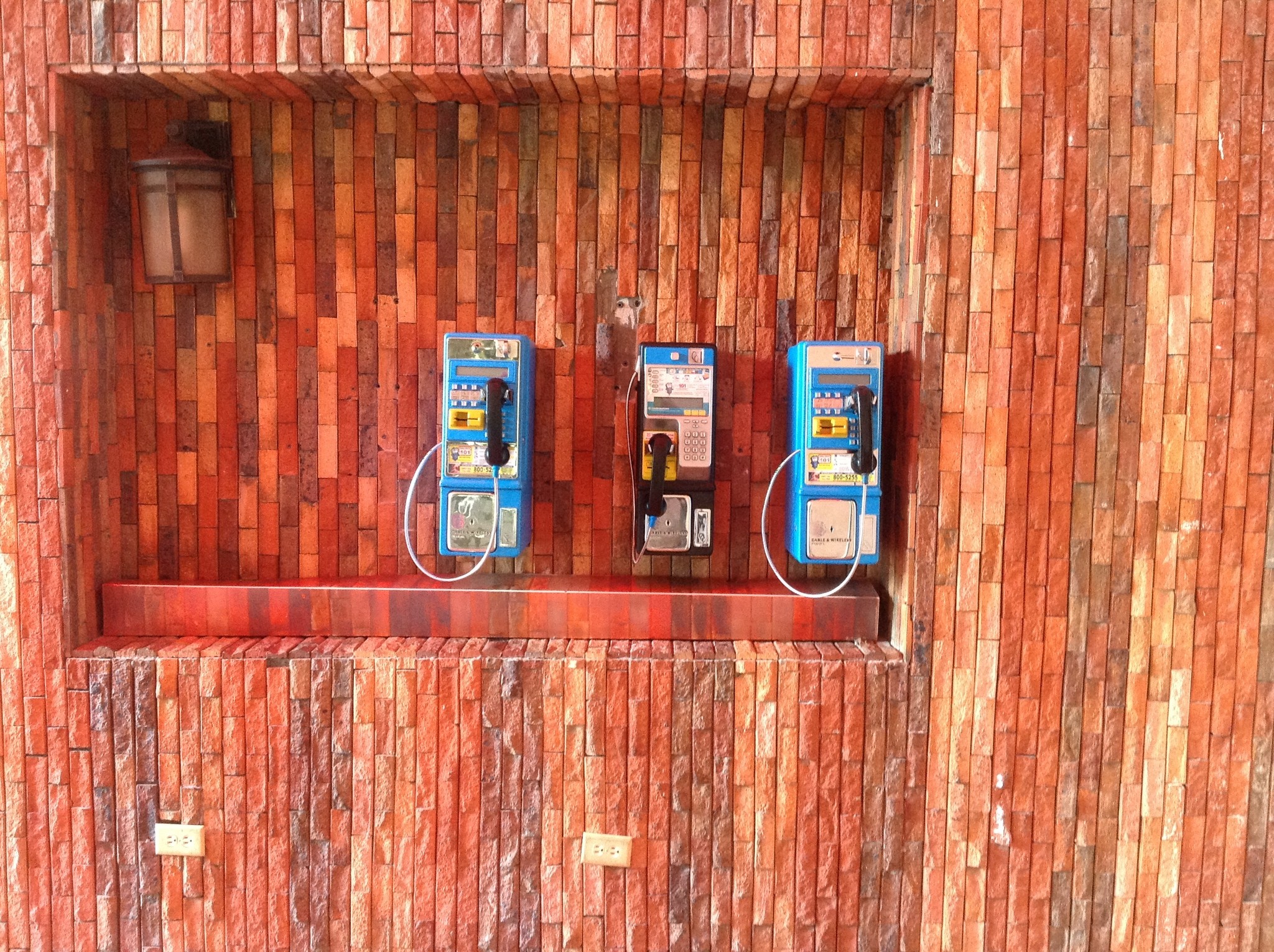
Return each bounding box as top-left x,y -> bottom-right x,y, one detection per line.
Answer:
130,121 -> 233,284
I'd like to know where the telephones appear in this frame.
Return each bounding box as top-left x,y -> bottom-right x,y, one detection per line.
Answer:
439,333 -> 531,492
787,340 -> 881,496
635,340 -> 717,519
